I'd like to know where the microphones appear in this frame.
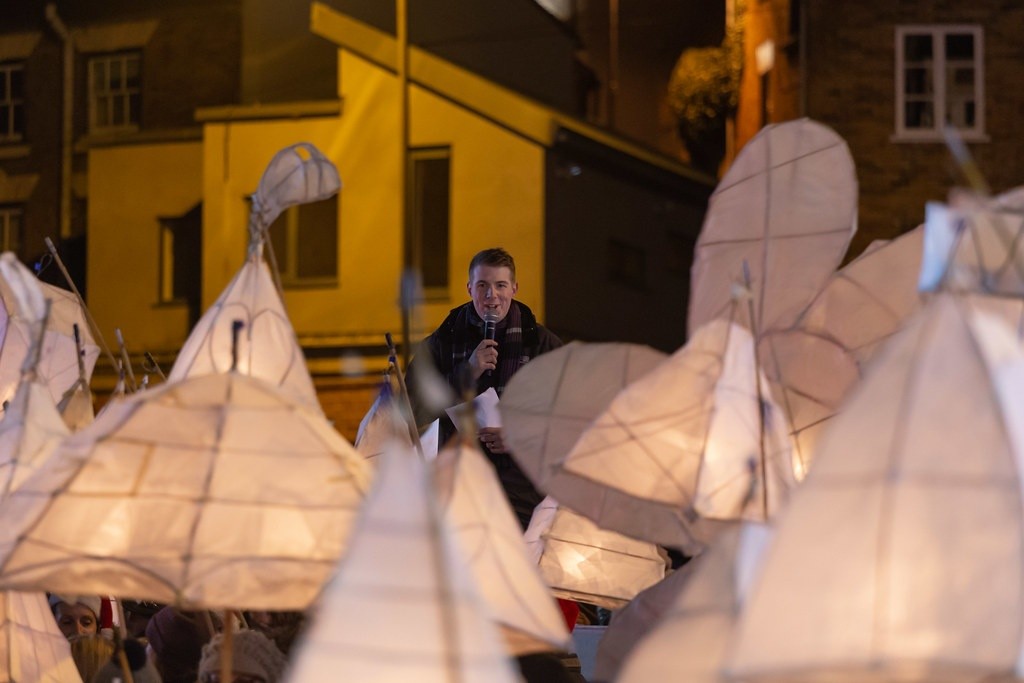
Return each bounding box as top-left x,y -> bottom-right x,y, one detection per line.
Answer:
481,309 -> 500,377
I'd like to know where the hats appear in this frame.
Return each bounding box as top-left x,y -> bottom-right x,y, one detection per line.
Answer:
197,630 -> 287,683
47,593 -> 101,621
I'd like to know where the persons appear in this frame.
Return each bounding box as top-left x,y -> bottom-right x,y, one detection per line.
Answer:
404,246 -> 566,532
43,591 -> 304,683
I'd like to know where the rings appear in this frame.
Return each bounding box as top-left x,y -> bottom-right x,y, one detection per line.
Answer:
491,442 -> 495,448
